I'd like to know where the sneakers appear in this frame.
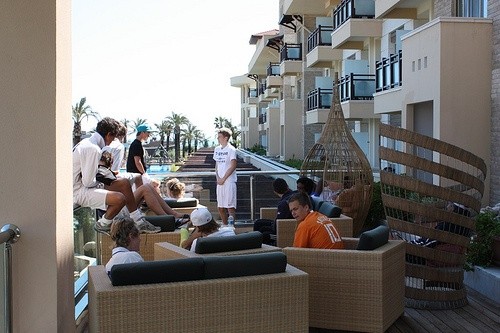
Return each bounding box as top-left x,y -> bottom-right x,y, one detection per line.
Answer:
94,220 -> 113,237
134,216 -> 161,234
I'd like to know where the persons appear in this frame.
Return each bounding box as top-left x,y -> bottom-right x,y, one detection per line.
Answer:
72,117 -> 161,237
181,208 -> 235,251
167,178 -> 184,198
254,178 -> 293,230
212,127 -> 237,225
99,149 -> 190,228
106,218 -> 145,274
258,176 -> 317,245
127,124 -> 152,194
97,126 -> 149,210
287,192 -> 346,249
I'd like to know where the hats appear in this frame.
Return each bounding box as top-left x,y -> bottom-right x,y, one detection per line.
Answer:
137,124 -> 157,133
190,208 -> 212,226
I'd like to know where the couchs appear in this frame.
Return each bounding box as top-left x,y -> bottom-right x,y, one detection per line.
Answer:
282,225 -> 406,333
87,189 -> 310,333
260,194 -> 354,248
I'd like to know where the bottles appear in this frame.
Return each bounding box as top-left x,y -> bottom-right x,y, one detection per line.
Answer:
228,213 -> 235,230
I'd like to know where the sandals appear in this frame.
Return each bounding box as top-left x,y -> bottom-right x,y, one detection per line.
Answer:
175,218 -> 190,228
179,214 -> 190,218
